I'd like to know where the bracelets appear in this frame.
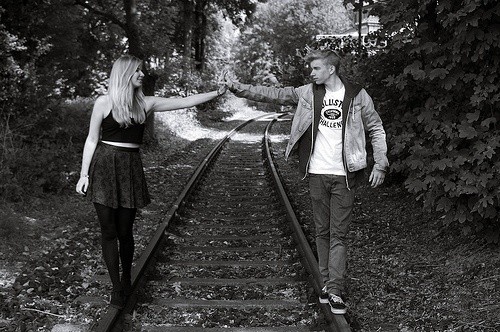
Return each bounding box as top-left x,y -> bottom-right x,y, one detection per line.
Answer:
78,174 -> 90,177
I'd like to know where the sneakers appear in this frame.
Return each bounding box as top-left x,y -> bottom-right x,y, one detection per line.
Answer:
319,287 -> 329,304
327,294 -> 347,314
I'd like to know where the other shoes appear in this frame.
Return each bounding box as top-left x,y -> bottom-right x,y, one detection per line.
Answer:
110,288 -> 137,309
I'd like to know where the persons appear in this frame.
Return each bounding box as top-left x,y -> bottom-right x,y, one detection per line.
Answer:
219,49 -> 392,314
70,52 -> 229,309
